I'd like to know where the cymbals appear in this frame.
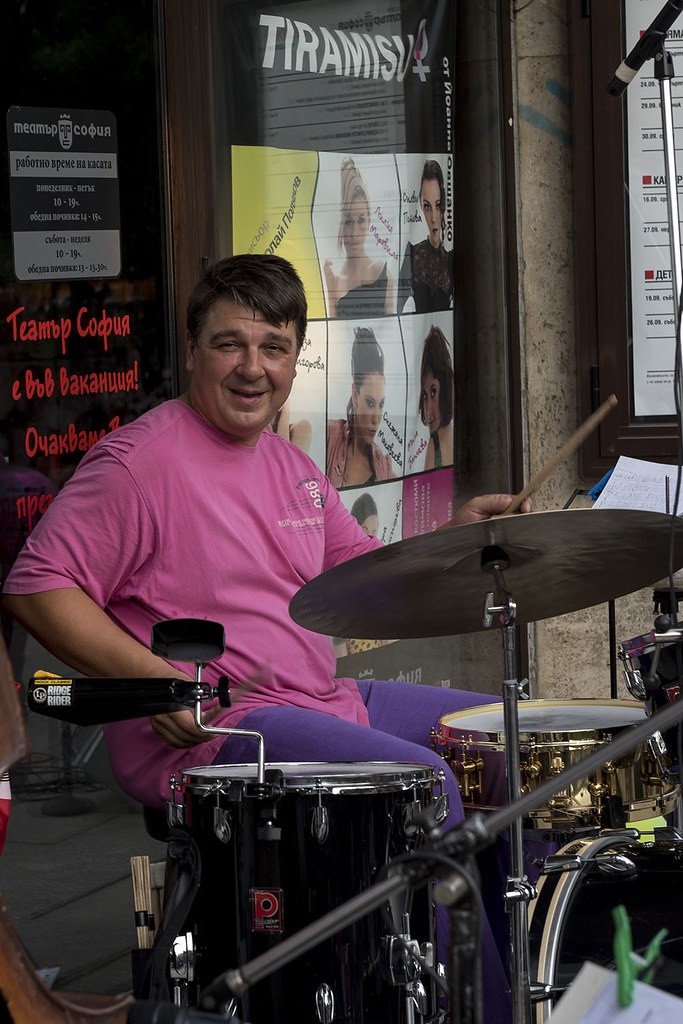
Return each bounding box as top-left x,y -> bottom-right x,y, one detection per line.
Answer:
287,506 -> 683,641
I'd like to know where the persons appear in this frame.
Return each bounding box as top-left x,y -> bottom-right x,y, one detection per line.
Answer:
5,252 -> 553,1024
268,399 -> 312,454
399,159 -> 453,313
323,154 -> 396,317
419,325 -> 453,472
347,493 -> 392,655
328,327 -> 399,486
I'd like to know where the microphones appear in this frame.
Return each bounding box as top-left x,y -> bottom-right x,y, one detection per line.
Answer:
606,0 -> 683,98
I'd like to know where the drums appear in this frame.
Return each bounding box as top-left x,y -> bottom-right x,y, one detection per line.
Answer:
142,620 -> 683,1024
